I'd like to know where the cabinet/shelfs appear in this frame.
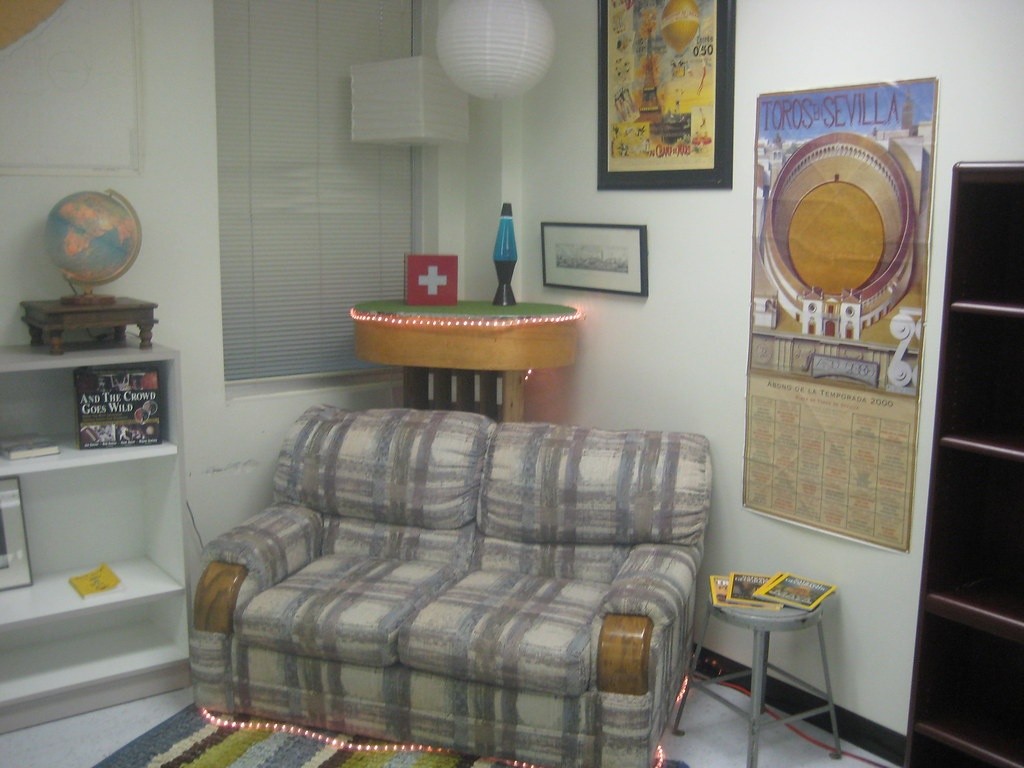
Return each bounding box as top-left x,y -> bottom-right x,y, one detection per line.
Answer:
904,159 -> 1024,768
0,330 -> 194,735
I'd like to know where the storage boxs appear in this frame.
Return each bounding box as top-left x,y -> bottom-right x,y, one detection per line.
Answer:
71,368 -> 163,451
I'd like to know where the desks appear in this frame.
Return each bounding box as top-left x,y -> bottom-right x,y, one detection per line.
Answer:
673,591 -> 842,768
350,300 -> 583,422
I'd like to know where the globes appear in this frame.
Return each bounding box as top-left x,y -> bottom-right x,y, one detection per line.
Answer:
45,188 -> 142,305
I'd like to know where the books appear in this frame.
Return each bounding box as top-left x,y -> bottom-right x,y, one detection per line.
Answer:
0,433 -> 60,459
73,366 -> 162,450
710,572 -> 836,611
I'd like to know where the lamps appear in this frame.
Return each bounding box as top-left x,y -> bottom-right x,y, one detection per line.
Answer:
435,0 -> 556,103
349,0 -> 468,147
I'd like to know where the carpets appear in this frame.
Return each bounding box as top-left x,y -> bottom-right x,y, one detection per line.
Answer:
93,703 -> 687,766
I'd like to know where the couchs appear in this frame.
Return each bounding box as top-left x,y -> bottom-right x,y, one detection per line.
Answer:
188,401 -> 715,767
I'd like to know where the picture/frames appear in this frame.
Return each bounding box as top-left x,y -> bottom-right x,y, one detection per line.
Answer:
1,475 -> 34,592
541,222 -> 648,298
598,0 -> 737,189
